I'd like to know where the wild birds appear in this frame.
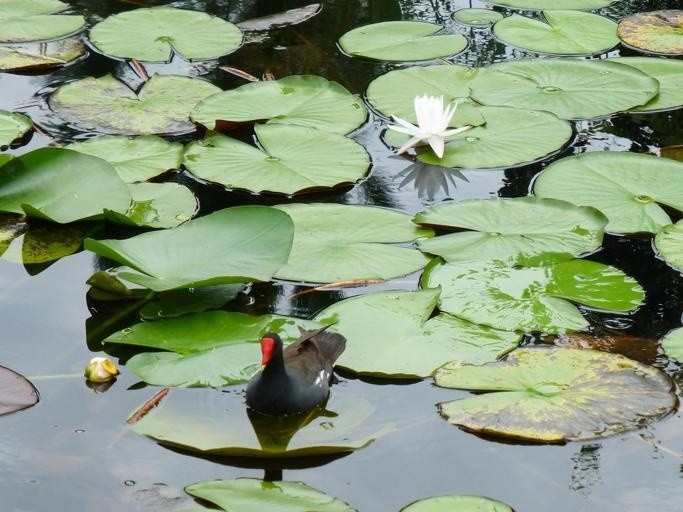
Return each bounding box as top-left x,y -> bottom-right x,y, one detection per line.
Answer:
244,321 -> 347,417
